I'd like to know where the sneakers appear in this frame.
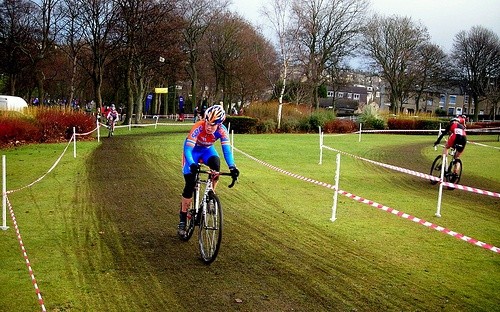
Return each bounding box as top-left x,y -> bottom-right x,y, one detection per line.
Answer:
178,222 -> 187,236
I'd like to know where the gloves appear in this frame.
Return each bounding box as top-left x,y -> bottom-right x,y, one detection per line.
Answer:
230,168 -> 239,179
191,163 -> 201,174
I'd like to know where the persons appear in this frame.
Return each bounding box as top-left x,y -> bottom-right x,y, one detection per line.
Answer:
178,105 -> 238,236
433,114 -> 468,180
106,107 -> 119,138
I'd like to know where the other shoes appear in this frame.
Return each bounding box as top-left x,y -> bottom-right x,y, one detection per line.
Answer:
111,131 -> 114,136
452,173 -> 460,178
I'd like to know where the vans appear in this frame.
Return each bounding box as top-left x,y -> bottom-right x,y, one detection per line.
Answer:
0,95 -> 29,113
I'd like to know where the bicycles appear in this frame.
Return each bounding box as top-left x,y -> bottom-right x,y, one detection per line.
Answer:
177,160 -> 238,265
430,143 -> 464,190
107,118 -> 113,139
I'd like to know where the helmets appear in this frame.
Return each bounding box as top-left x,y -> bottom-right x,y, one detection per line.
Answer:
450,118 -> 460,123
462,114 -> 466,118
204,105 -> 226,126
